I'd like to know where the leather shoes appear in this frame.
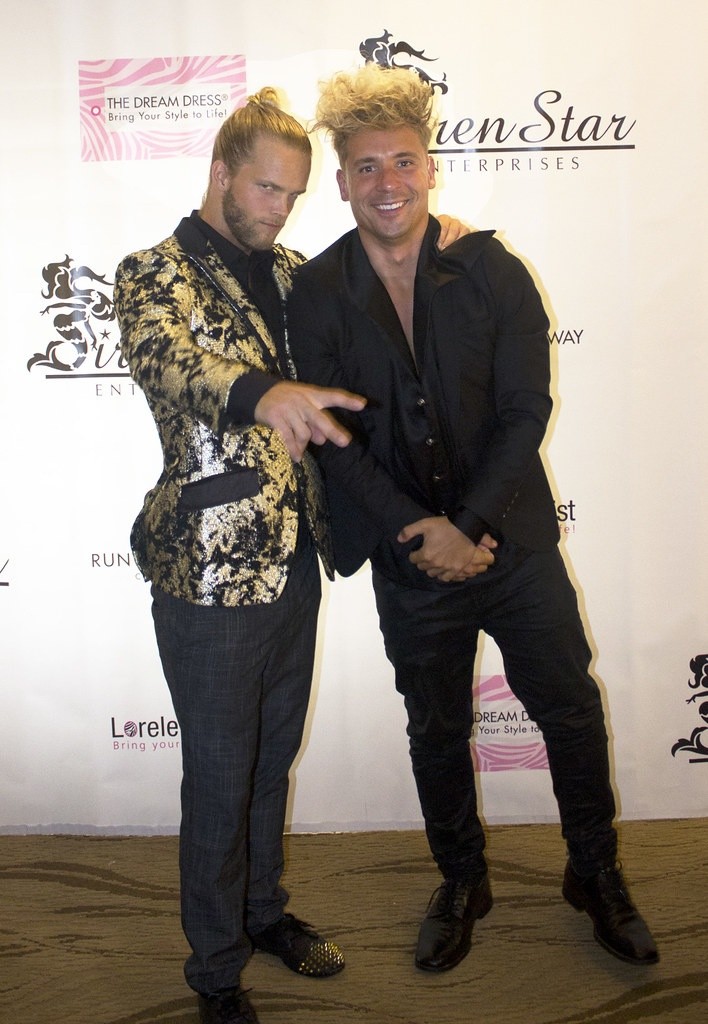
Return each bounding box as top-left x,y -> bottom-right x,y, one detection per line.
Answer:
562,841 -> 660,966
254,911 -> 345,978
198,988 -> 260,1023
412,859 -> 493,972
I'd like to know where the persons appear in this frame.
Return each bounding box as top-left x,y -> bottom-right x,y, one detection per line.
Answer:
115,87 -> 470,1024
291,65 -> 661,976
